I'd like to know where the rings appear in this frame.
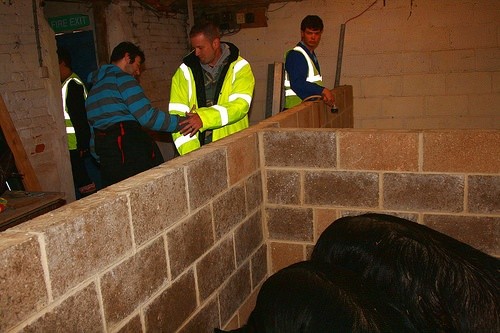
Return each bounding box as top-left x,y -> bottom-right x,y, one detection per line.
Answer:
190,126 -> 194,131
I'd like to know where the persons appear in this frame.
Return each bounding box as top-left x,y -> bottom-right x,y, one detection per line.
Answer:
168,23 -> 255,156
58,52 -> 100,192
84,41 -> 191,184
283,15 -> 335,113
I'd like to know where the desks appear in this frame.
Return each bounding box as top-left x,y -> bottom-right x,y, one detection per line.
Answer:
0,190 -> 66,232
